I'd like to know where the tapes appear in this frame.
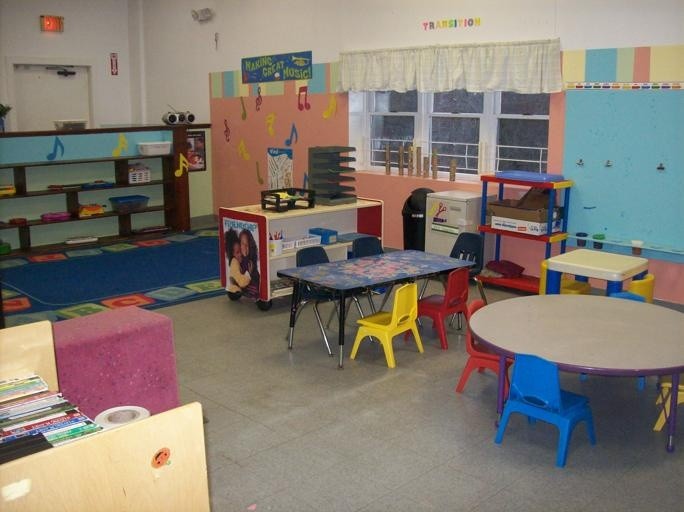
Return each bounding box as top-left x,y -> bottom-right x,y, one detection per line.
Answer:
94,405 -> 150,429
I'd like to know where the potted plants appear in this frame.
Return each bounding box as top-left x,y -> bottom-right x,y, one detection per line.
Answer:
0,103 -> 13,132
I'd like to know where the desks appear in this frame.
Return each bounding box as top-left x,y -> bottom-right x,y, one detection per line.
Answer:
278,249 -> 476,371
469,294 -> 684,453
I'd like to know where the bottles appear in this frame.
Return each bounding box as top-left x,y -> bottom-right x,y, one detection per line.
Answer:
575,233 -> 587,246
592,234 -> 604,249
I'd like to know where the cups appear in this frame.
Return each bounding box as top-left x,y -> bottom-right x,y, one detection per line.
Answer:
632,240 -> 643,254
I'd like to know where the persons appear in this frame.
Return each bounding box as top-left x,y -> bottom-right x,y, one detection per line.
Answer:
223,227 -> 253,294
227,229 -> 260,297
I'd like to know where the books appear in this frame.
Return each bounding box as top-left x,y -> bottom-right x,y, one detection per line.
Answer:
1,371 -> 107,464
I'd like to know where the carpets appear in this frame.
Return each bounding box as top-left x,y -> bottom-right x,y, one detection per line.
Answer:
0,227 -> 227,328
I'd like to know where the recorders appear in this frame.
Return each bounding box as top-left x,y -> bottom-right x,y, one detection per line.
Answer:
161,111 -> 195,124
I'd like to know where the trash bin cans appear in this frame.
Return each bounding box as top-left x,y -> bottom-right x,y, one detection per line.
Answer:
402,188 -> 434,252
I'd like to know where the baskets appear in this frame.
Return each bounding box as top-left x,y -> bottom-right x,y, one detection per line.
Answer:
128,171 -> 151,184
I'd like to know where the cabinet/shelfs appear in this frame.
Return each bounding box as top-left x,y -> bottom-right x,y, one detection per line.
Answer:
0,126 -> 190,262
475,176 -> 573,294
218,205 -> 383,310
